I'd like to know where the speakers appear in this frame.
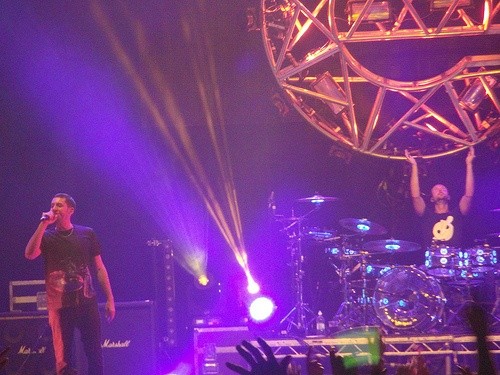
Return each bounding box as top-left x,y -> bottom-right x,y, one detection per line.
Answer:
0,310 -> 58,375
99,300 -> 158,375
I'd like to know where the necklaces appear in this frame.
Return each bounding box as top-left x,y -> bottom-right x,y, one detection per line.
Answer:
55,224 -> 74,238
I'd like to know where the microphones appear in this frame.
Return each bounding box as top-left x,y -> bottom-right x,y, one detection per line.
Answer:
392,292 -> 408,299
40,215 -> 50,221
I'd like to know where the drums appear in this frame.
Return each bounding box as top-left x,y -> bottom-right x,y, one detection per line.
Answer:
427,245 -> 463,277
463,246 -> 497,277
372,265 -> 445,333
445,271 -> 482,284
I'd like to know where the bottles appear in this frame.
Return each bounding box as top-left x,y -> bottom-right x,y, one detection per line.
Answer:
316,309 -> 326,335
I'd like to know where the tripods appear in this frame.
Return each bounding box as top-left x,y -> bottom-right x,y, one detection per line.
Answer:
440,281 -> 500,332
281,202 -> 376,338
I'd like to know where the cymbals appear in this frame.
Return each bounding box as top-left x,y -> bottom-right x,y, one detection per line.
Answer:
361,239 -> 423,254
488,209 -> 500,212
339,218 -> 388,237
296,195 -> 339,202
487,233 -> 500,237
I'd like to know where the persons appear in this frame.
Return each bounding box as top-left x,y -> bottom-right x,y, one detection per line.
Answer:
224,300 -> 498,375
404,146 -> 475,255
24,193 -> 115,375
